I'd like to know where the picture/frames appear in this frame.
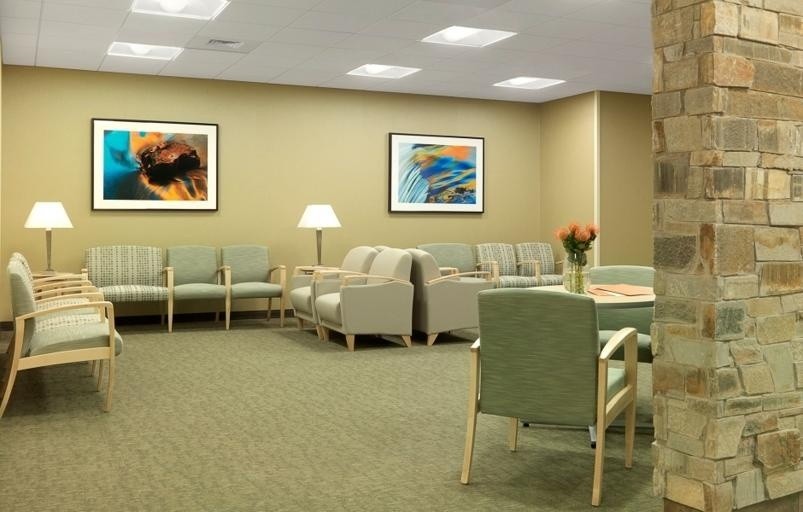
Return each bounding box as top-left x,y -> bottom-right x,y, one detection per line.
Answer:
387,132 -> 484,214
89,116 -> 220,212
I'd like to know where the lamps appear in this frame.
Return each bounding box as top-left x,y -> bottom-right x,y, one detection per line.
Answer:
296,203 -> 340,266
23,200 -> 75,270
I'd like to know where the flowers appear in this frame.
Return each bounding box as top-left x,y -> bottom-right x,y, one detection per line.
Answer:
552,220 -> 600,293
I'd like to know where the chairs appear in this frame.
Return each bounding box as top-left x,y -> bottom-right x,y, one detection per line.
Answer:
459,289 -> 638,508
518,265 -> 655,428
289,242 -> 655,352
83,245 -> 287,334
1,252 -> 122,418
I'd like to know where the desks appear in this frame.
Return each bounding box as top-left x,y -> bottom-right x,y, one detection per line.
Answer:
523,283 -> 656,448
